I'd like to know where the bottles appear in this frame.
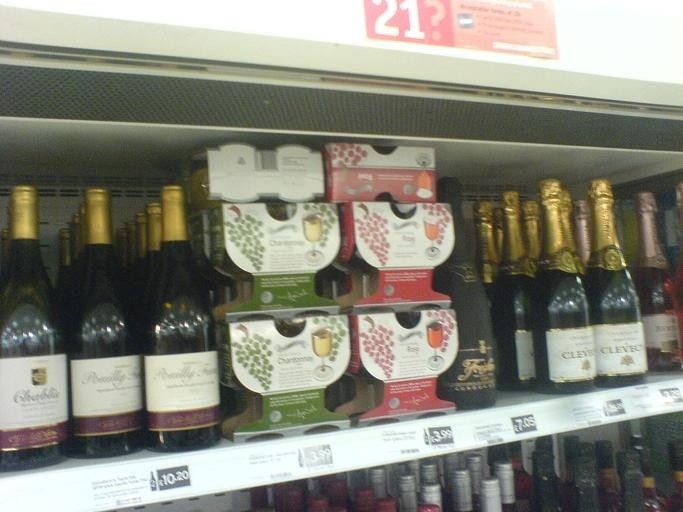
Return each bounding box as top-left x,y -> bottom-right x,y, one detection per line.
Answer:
432,177 -> 497,418
248,418 -> 683,512
470,175 -> 682,394
0,177 -> 225,472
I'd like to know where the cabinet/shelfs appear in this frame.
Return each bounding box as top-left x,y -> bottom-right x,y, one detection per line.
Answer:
0,2 -> 683,512
